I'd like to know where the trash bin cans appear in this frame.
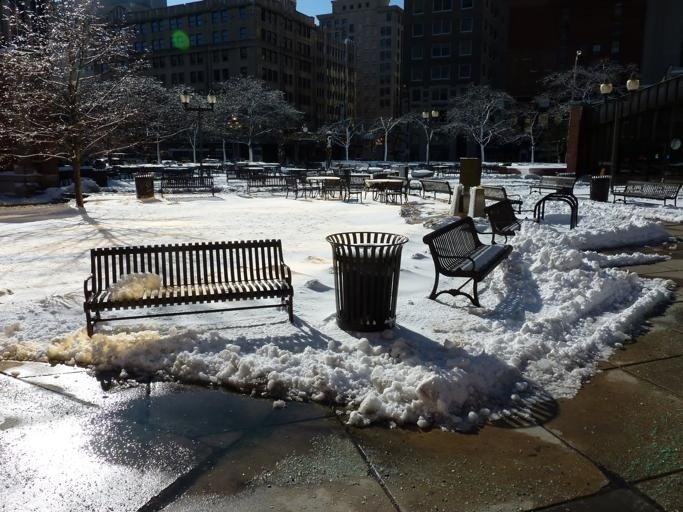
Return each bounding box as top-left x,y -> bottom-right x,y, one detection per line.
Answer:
325,232 -> 409,332
591,176 -> 611,202
134,175 -> 154,199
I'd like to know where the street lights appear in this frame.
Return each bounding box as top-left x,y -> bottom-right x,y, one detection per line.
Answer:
598,72 -> 640,194
179,88 -> 216,185
422,107 -> 438,168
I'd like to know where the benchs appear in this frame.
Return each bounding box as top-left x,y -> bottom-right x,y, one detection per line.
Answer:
481,184 -> 523,246
529,175 -> 577,194
422,216 -> 513,307
82,238 -> 296,339
611,181 -> 683,207
